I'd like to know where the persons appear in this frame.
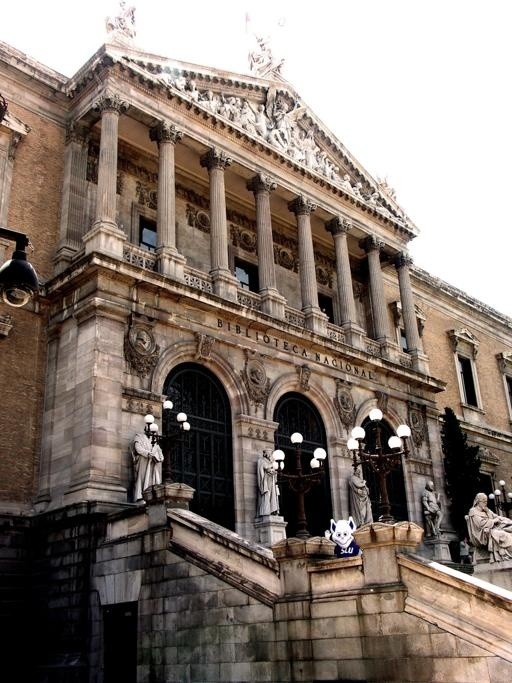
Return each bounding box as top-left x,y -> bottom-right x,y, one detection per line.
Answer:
466,491 -> 512,563
348,466 -> 374,528
130,423 -> 165,502
153,67 -> 387,212
256,446 -> 281,517
421,479 -> 443,537
